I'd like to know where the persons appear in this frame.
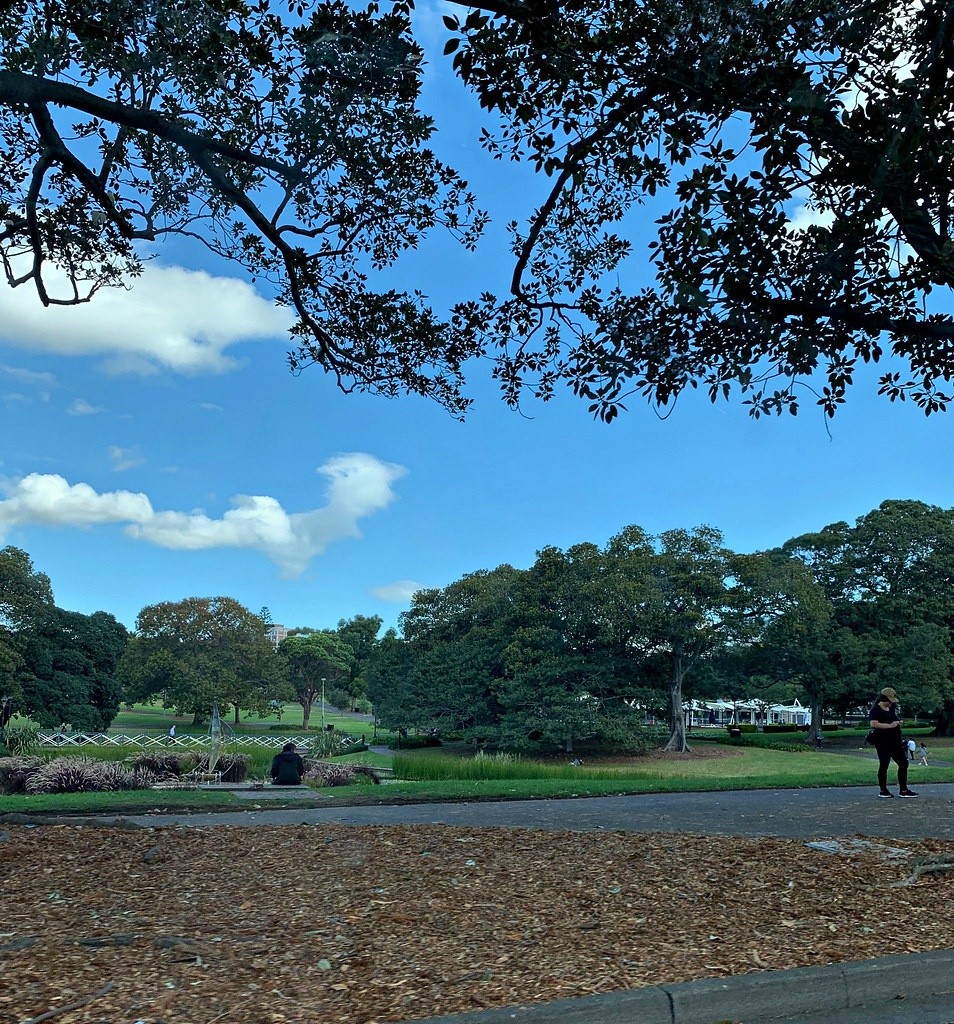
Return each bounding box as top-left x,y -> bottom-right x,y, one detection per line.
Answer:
271,743 -> 307,786
869,686 -> 919,799
61,726 -> 67,741
908,737 -> 916,760
915,743 -> 929,767
165,725 -> 177,745
902,740 -> 909,759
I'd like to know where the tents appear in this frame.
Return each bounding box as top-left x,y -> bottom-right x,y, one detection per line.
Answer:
643,699 -> 811,727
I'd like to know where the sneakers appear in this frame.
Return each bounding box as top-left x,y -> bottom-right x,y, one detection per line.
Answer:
898,790 -> 919,798
878,790 -> 894,797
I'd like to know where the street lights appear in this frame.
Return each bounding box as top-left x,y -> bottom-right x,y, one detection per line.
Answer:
320,678 -> 326,749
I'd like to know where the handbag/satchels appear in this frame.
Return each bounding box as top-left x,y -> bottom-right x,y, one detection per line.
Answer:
863,730 -> 874,745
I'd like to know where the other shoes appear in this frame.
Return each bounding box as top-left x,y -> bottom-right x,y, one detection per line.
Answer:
918,763 -> 922,766
926,764 -> 929,767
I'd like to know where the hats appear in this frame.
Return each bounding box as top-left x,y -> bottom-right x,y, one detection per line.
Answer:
881,687 -> 899,702
